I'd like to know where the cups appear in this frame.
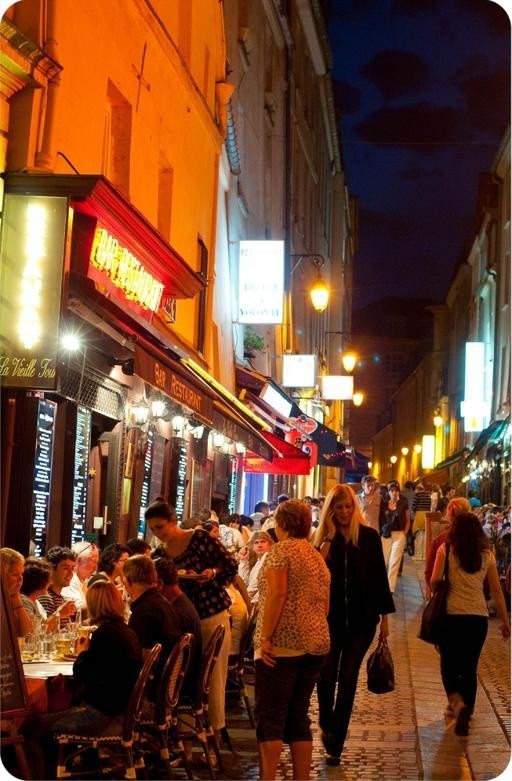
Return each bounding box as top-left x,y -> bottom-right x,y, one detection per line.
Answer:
20,609 -> 93,663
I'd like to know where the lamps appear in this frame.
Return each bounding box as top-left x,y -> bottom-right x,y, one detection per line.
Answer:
106,355 -> 135,376
291,252 -> 331,313
324,331 -> 360,375
131,394 -> 224,452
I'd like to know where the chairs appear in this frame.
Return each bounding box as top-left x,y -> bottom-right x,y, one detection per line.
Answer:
0,708 -> 33,779
225,606 -> 258,732
132,629 -> 195,781
51,642 -> 168,781
173,624 -> 229,775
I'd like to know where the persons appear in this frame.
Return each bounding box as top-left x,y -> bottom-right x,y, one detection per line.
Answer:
0,476 -> 510,781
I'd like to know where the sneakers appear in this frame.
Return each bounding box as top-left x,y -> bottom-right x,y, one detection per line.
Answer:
444,704 -> 473,736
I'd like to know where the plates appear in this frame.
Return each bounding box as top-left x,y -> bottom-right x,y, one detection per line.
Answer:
177,572 -> 207,579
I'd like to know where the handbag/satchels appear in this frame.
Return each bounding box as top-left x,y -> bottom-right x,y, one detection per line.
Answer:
367,633 -> 394,694
416,580 -> 451,646
